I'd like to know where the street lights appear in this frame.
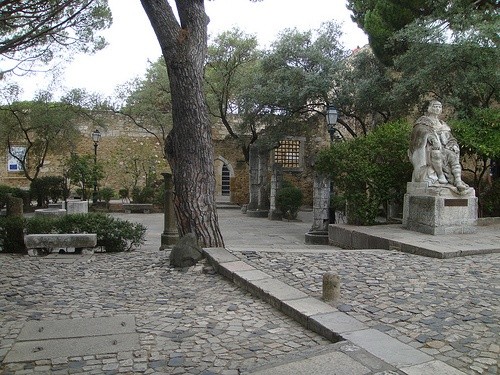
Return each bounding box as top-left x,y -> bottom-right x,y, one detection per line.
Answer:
91,127 -> 101,203
326,102 -> 338,224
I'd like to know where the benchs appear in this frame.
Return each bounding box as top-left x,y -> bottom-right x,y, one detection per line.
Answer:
23,233 -> 97,256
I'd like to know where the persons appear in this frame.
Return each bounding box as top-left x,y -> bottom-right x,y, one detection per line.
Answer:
408,100 -> 466,192
72,179 -> 83,199
